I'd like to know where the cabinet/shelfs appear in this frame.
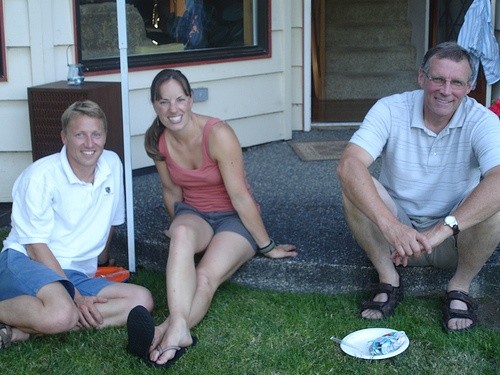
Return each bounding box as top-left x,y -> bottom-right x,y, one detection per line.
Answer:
27,81 -> 125,175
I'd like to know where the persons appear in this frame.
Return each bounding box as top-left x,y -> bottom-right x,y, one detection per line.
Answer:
127,69 -> 298,366
0,101 -> 153,346
337,42 -> 500,332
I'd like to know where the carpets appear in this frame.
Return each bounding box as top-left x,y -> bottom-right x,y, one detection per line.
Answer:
289,138 -> 383,162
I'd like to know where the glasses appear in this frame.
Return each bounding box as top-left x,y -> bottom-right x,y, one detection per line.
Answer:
423,70 -> 467,89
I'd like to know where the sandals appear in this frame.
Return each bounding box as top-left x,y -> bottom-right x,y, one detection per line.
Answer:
0,324 -> 44,349
359,276 -> 404,321
442,288 -> 479,333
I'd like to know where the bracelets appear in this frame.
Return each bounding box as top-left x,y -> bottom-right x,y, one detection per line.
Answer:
258,239 -> 275,254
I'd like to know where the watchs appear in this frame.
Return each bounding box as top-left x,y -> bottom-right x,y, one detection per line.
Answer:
444,216 -> 460,236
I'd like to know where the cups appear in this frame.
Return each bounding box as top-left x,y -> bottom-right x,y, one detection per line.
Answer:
68,65 -> 85,85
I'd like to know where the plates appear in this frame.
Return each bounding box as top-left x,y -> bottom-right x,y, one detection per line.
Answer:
340,328 -> 410,359
96,267 -> 130,283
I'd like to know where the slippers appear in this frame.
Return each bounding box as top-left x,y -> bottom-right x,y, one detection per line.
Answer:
127,304 -> 154,359
144,334 -> 198,368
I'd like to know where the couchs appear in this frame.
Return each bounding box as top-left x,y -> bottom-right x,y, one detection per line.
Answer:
80,1 -> 154,60
